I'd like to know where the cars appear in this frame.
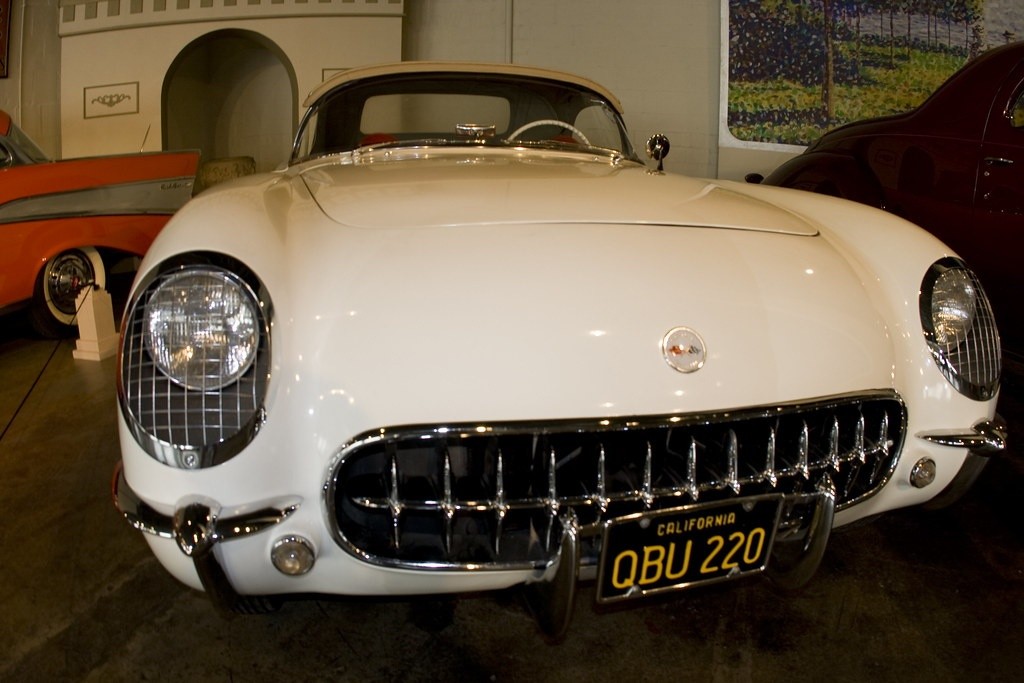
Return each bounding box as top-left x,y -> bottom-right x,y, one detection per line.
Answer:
114,60 -> 1008,648
0,111 -> 205,341
755,39 -> 1024,363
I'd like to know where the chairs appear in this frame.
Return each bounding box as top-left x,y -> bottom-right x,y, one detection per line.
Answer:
357,133 -> 399,148
